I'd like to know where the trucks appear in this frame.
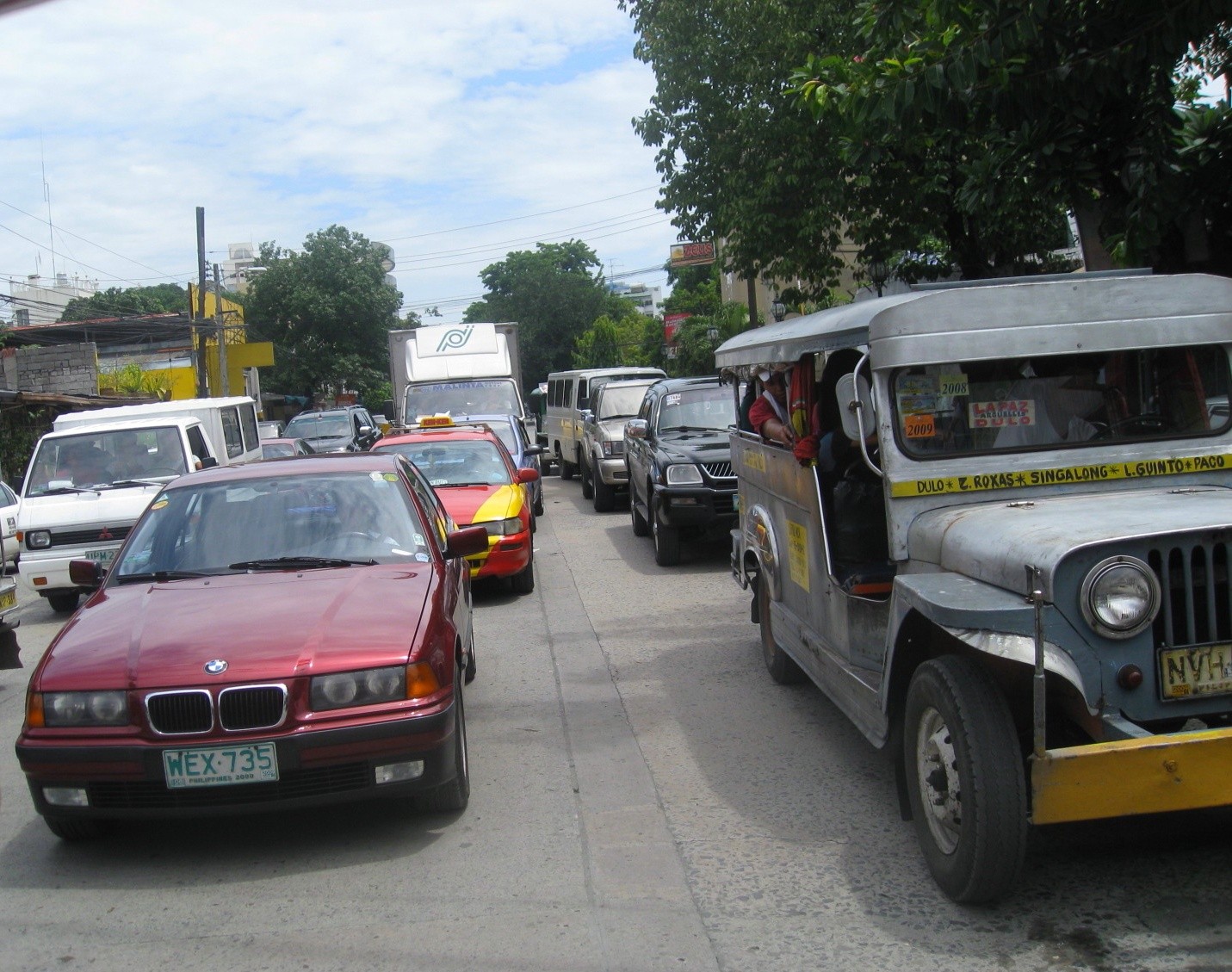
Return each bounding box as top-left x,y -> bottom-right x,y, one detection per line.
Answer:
13,396 -> 265,613
387,322 -> 538,434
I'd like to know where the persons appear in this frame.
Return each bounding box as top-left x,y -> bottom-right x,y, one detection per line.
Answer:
301,496 -> 398,556
441,448 -> 509,482
728,345 -> 1141,594
30,430 -> 205,484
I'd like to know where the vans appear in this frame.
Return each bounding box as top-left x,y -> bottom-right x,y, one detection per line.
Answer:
712,263 -> 1232,910
527,383 -> 560,477
547,366 -> 668,482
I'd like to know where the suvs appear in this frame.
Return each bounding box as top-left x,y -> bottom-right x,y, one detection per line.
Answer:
578,376 -> 669,513
622,374 -> 748,567
282,405 -> 383,454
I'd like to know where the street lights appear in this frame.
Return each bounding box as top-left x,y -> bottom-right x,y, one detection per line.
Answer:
198,266 -> 267,398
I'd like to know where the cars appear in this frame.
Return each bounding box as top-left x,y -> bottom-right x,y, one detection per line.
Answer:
0,481 -> 21,571
15,452 -> 489,849
408,414 -> 543,533
260,438 -> 315,461
258,420 -> 287,440
371,413 -> 396,435
369,416 -> 540,595
